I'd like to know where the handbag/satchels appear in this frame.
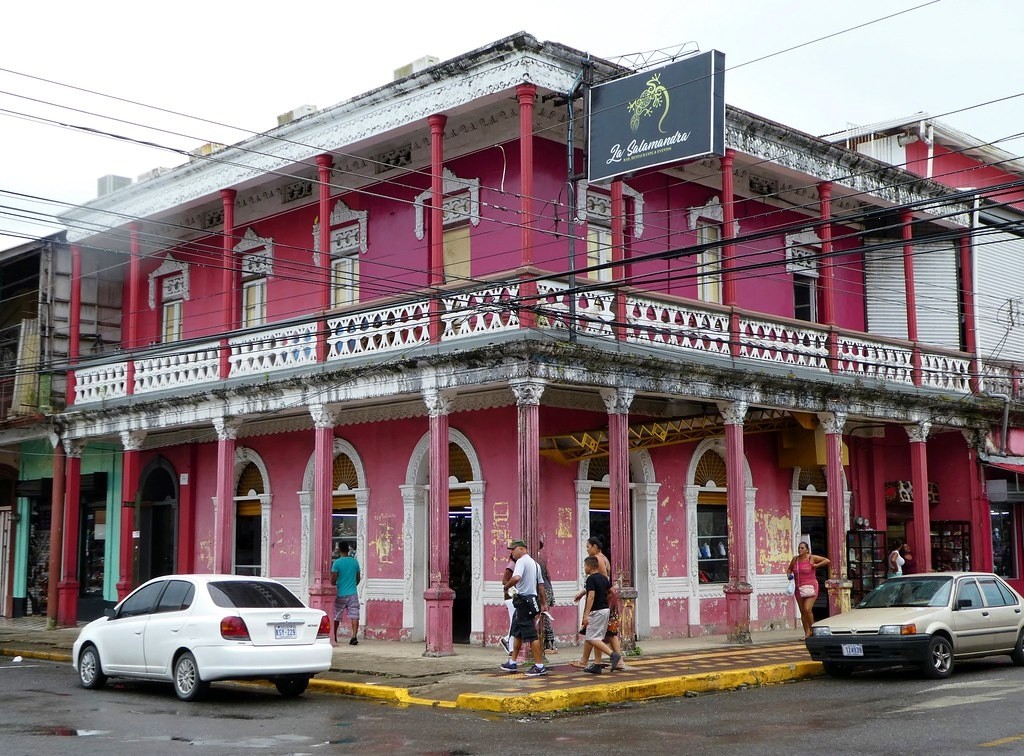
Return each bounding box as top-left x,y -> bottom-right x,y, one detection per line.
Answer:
786,578 -> 795,596
522,595 -> 541,616
799,584 -> 815,598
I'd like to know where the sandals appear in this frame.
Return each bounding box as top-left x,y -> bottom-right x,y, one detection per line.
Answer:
569,660 -> 587,668
605,662 -> 625,670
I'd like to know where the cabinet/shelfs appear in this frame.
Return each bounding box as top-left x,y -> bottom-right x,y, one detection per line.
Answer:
930,520 -> 972,572
847,531 -> 887,602
697,512 -> 728,582
80,504 -> 107,595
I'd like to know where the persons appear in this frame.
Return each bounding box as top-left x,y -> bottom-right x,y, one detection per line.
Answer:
900,543 -> 917,576
579,555 -> 621,674
570,536 -> 627,668
328,541 -> 361,645
786,541 -> 832,640
537,540 -> 557,654
500,550 -> 531,654
887,539 -> 906,578
495,538 -> 549,676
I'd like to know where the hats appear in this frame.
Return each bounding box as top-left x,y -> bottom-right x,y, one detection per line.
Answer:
507,540 -> 526,549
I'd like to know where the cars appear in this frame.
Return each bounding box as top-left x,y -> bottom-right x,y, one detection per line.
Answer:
71,573 -> 333,700
807,573 -> 1023,680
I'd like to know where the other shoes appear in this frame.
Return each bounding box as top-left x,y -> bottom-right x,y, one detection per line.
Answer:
500,638 -> 509,653
545,648 -> 558,654
800,635 -> 808,641
350,637 -> 358,645
610,653 -> 621,672
583,663 -> 601,674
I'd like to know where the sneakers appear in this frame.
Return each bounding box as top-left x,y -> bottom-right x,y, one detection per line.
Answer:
500,661 -> 517,672
524,664 -> 547,676
